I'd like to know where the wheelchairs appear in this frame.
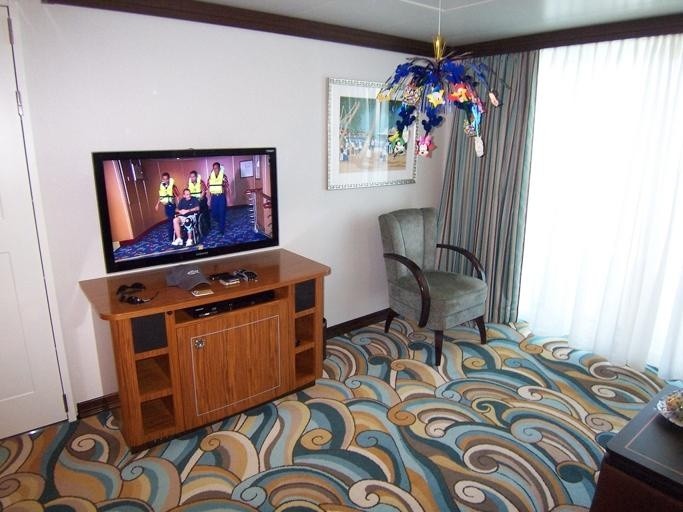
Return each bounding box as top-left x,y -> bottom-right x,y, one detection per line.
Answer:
172,198 -> 206,245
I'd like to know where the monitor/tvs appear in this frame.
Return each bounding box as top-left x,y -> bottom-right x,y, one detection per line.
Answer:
92,148 -> 278,275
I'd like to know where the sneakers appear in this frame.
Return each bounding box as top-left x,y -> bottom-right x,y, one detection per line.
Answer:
171,239 -> 183,246
186,238 -> 194,247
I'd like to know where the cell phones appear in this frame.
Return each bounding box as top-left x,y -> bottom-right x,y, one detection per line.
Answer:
235,269 -> 257,281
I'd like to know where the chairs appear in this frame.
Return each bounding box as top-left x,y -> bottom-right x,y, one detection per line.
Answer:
378,207 -> 492,368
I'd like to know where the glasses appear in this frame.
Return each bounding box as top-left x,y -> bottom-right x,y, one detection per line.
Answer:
116,283 -> 145,295
119,291 -> 159,305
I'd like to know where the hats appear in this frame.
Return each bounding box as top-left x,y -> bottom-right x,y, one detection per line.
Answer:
166,264 -> 212,291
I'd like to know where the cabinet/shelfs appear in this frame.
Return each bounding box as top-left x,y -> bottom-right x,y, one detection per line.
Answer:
76,248 -> 331,452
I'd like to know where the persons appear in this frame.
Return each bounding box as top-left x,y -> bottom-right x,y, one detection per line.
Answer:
187,171 -> 211,230
207,162 -> 230,238
171,189 -> 200,247
154,172 -> 181,243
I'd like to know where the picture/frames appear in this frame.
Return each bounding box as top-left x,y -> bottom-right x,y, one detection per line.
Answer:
325,78 -> 421,190
